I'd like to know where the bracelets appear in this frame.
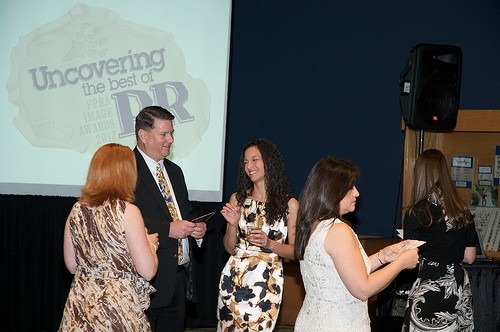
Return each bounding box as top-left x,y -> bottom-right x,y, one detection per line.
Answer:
378,249 -> 385,265
151,242 -> 156,252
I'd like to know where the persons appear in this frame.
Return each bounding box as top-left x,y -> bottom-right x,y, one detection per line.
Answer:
294,157 -> 419,332
57,143 -> 159,332
401,149 -> 477,332
130,106 -> 207,332
217,138 -> 300,332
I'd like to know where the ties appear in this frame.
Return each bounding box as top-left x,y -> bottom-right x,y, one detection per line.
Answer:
156,163 -> 184,264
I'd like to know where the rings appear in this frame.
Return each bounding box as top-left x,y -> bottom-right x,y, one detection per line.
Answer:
417,261 -> 419,263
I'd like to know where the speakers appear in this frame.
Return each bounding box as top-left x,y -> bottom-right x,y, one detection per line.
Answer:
399,43 -> 463,131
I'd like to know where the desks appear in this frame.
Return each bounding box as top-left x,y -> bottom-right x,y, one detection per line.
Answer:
277,238 -> 398,326
375,261 -> 500,332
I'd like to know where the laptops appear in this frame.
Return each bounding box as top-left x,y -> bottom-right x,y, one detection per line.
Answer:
473,228 -> 495,262
